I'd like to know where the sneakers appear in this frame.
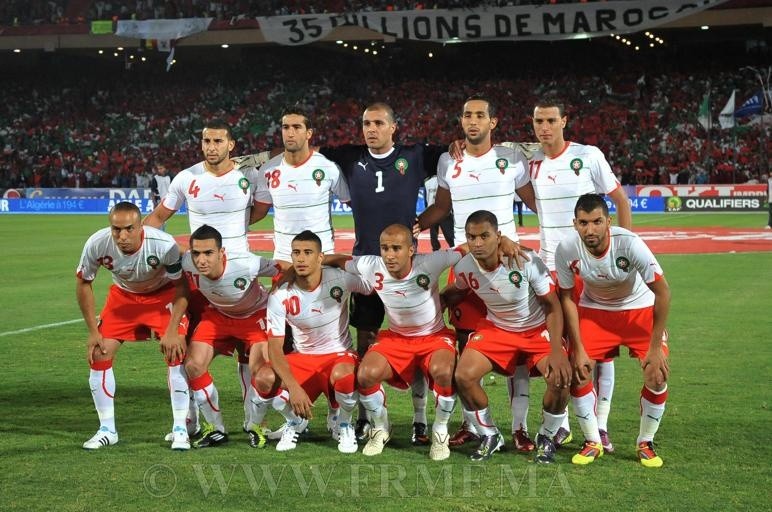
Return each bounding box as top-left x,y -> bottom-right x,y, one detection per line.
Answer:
82,426 -> 118,449
332,422 -> 358,453
510,429 -> 534,451
411,422 -> 428,446
449,425 -> 481,445
470,434 -> 505,462
164,418 -> 201,441
171,424 -> 191,449
551,427 -> 573,448
268,423 -> 308,440
355,419 -> 370,444
276,417 -> 309,451
192,424 -> 229,448
243,421 -> 272,436
247,422 -> 267,449
428,431 -> 450,461
635,440 -> 663,467
362,424 -> 393,456
534,433 -> 556,465
571,441 -> 603,464
599,429 -> 615,454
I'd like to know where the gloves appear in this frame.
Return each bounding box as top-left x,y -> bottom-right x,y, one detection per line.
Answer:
500,141 -> 543,160
229,150 -> 271,169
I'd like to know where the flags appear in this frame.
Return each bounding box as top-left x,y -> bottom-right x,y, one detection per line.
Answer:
139,39 -> 176,64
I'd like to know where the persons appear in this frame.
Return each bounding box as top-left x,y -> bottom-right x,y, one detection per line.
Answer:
268,221 -> 533,461
309,101 -> 448,446
151,120 -> 250,172
491,97 -> 538,146
417,91 -> 538,451
439,210 -> 573,467
139,120 -> 260,442
1,77 -> 151,188
76,202 -> 199,450
553,193 -> 672,469
249,106 -> 422,441
151,162 -> 172,208
151,78 -> 309,120
250,230 -> 377,453
419,96 -> 463,147
180,225 -> 294,450
0,1 -> 515,27
250,120 -> 282,154
310,78 -> 417,147
564,68 -> 771,185
419,78 -> 563,96
447,95 -> 632,456
